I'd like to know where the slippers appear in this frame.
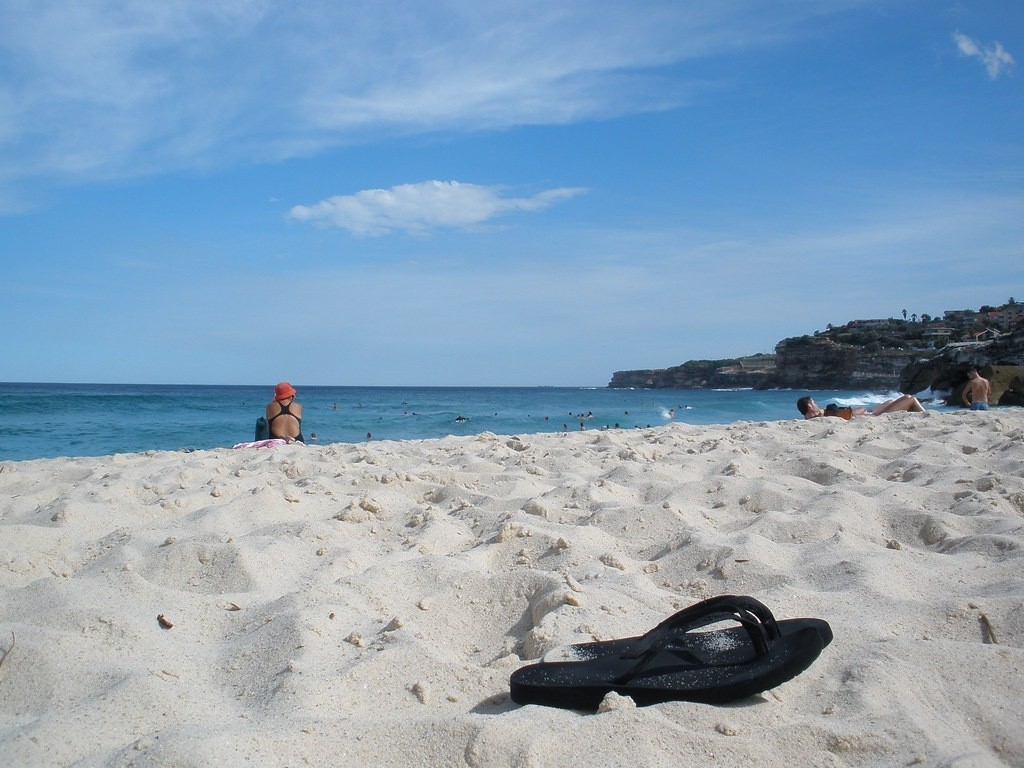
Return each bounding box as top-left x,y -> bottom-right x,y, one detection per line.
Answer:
510,594 -> 832,709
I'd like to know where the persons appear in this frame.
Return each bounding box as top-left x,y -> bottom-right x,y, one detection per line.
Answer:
266,382 -> 304,443
796,394 -> 926,420
310,398 -> 688,440
961,367 -> 991,410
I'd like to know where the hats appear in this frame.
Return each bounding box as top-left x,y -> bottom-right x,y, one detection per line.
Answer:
275,382 -> 296,401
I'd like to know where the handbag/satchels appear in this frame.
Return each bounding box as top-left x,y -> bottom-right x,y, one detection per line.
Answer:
824,404 -> 852,420
